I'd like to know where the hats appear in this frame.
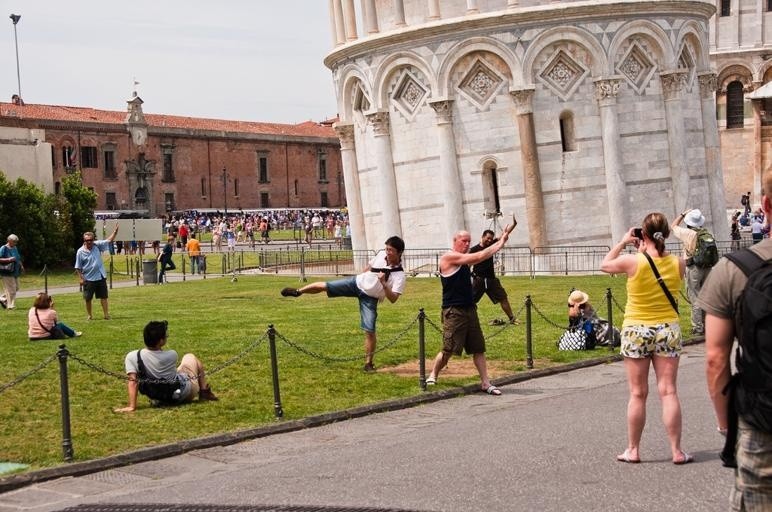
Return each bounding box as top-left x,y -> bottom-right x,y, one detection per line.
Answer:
684,209 -> 705,227
568,291 -> 588,305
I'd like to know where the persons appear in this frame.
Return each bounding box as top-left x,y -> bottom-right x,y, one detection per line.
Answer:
671,208 -> 718,334
112,321 -> 219,414
281,235 -> 407,376
599,214 -> 693,462
568,289 -> 600,330
97,205 -> 352,284
0,232 -> 26,309
469,215 -> 518,326
695,175 -> 771,512
74,220 -> 120,319
27,291 -> 83,338
427,223 -> 510,396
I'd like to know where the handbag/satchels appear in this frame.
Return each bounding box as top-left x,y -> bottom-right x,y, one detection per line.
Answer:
558,329 -> 586,351
50,325 -> 61,337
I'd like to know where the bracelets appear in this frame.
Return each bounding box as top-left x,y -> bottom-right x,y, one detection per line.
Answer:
681,212 -> 686,217
622,240 -> 627,248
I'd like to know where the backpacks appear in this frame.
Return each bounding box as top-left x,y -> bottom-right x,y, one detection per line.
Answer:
685,228 -> 718,269
720,250 -> 772,468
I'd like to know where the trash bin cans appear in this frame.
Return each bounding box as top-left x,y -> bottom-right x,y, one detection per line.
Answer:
199,255 -> 206,272
143,259 -> 158,284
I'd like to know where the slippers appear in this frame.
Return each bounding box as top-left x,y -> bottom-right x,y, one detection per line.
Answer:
481,385 -> 501,396
617,449 -> 641,463
489,320 -> 505,325
673,450 -> 693,464
426,378 -> 437,385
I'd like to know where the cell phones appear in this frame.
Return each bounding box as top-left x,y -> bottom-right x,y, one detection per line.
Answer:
49,296 -> 51,301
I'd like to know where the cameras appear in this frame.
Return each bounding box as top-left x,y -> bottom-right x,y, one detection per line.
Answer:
634,228 -> 643,240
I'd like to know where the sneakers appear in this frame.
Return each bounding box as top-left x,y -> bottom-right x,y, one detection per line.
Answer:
0,300 -> 6,309
364,362 -> 377,374
281,287 -> 302,297
198,383 -> 218,401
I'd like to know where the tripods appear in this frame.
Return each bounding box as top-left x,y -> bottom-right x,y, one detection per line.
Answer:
489,219 -> 521,276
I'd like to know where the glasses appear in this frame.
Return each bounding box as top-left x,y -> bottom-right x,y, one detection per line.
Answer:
85,239 -> 94,241
